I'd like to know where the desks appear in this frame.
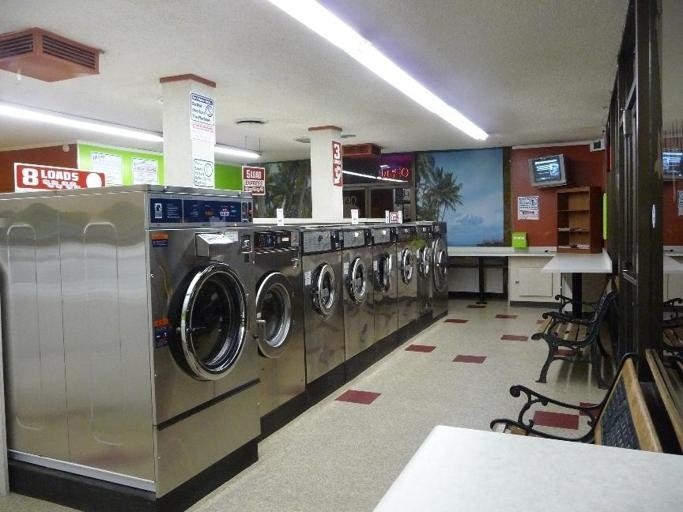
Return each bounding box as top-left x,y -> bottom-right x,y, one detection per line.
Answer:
662,252 -> 683,275
366,421 -> 682,512
445,249 -> 565,306
540,249 -> 613,318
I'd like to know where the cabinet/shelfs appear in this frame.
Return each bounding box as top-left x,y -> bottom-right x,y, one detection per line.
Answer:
556,185 -> 602,253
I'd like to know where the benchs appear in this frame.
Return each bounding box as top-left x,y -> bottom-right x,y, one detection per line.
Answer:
659,295 -> 682,355
533,274 -> 617,391
642,345 -> 682,454
489,347 -> 665,454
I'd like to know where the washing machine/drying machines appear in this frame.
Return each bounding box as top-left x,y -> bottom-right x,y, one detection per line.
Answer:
255,220 -> 450,419
1,181 -> 264,499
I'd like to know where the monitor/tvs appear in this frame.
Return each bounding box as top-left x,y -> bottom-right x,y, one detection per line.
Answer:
661,149 -> 682,180
528,153 -> 569,189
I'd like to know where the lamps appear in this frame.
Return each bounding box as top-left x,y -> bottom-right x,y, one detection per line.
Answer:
0,96 -> 262,161
